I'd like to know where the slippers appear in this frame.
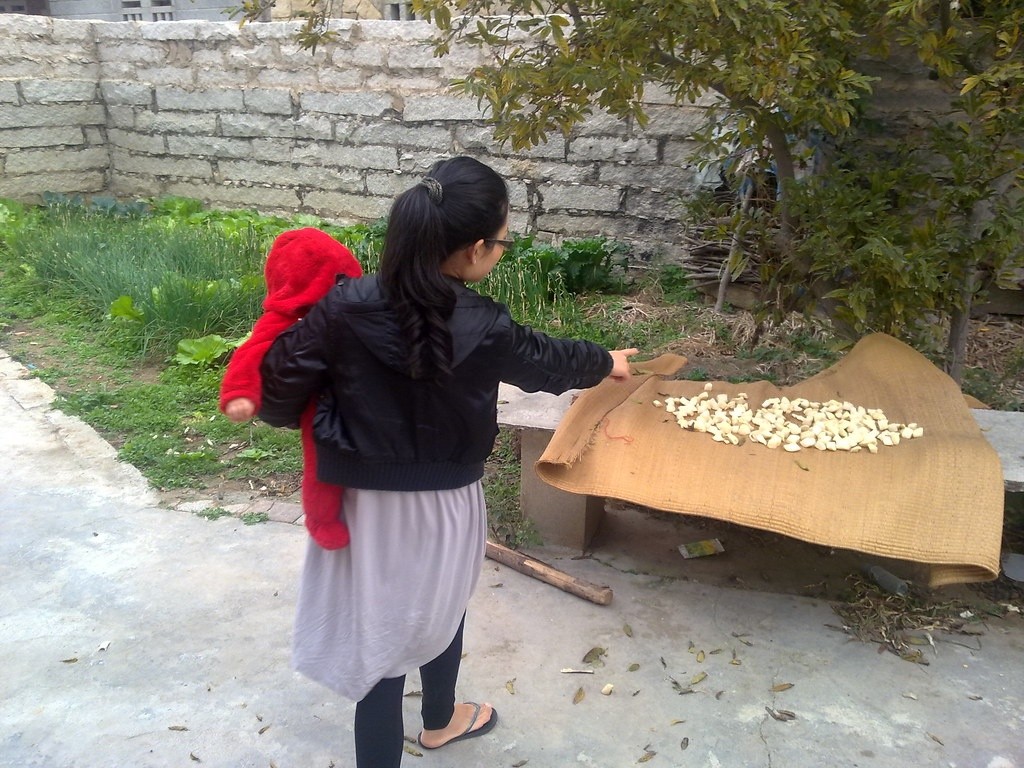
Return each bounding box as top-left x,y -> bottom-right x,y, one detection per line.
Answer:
418,702 -> 497,750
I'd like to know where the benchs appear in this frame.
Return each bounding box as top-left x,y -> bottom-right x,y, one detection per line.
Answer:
496,381 -> 1024,551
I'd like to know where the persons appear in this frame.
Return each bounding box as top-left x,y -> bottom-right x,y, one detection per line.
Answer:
260,156 -> 639,768
220,228 -> 364,550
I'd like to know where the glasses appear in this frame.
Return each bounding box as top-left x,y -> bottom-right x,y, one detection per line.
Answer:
462,232 -> 515,251
